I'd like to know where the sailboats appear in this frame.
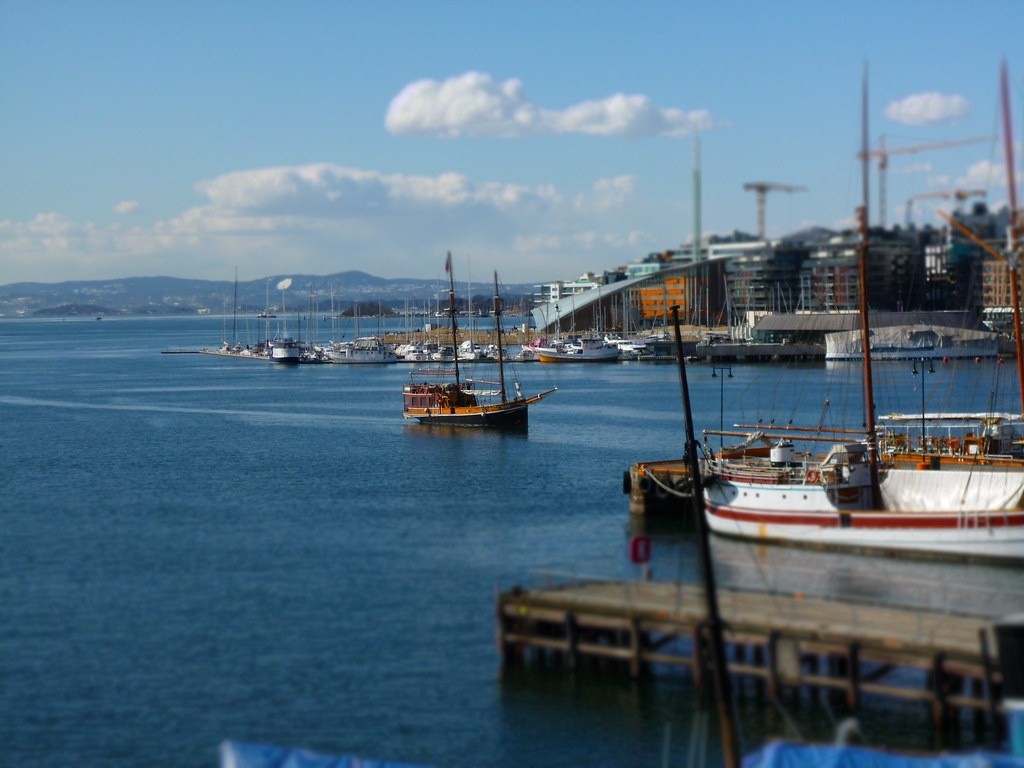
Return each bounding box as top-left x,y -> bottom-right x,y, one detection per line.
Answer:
401,253 -> 562,432
268,279 -> 645,369
619,57 -> 1024,570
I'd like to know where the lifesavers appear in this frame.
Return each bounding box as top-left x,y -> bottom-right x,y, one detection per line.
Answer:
806,469 -> 817,483
629,534 -> 653,564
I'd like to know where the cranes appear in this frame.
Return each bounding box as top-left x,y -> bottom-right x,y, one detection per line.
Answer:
855,132 -> 1001,229
740,180 -> 812,243
